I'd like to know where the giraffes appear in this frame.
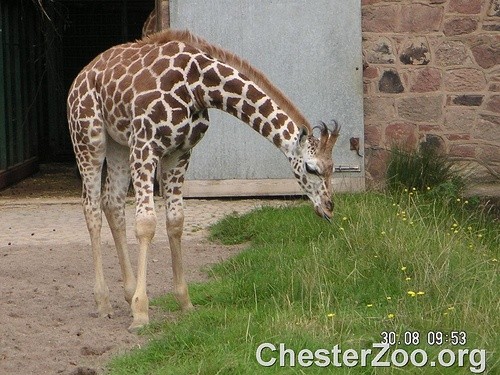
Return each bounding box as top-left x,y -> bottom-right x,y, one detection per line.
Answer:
66,28 -> 341,336
